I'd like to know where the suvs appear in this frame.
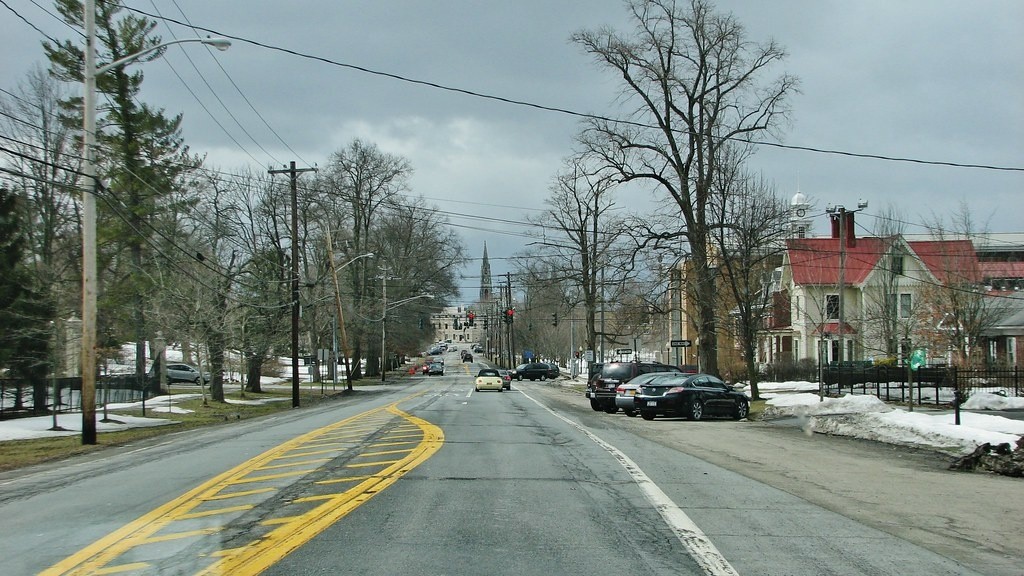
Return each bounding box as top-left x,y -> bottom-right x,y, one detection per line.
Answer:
506,363 -> 560,381
166,364 -> 210,385
586,360 -> 684,414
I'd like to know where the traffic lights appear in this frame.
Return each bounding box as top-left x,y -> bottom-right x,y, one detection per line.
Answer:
552,313 -> 558,327
502,309 -> 514,323
419,318 -> 423,329
483,320 -> 487,330
453,319 -> 458,330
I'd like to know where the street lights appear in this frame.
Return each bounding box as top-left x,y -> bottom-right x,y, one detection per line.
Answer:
333,253 -> 373,385
579,345 -> 582,375
83,39 -> 231,443
382,295 -> 434,381
469,313 -> 474,326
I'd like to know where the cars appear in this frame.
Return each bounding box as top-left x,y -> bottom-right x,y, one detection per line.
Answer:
615,372 -> 750,421
474,368 -> 511,393
471,343 -> 484,353
461,349 -> 473,363
423,356 -> 444,376
428,340 -> 457,354
